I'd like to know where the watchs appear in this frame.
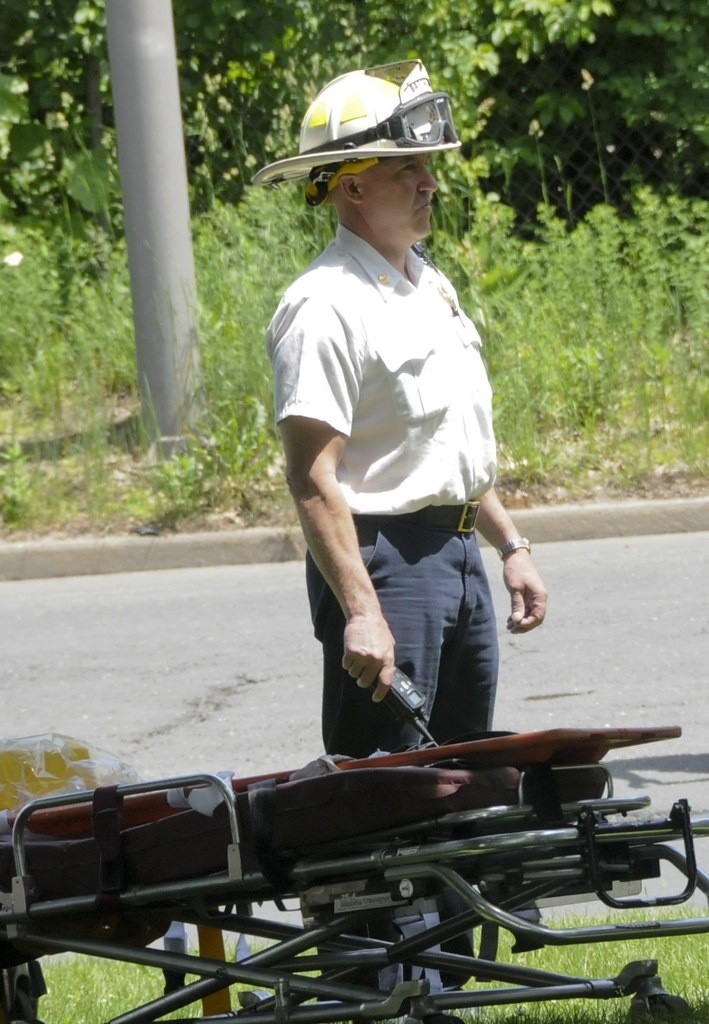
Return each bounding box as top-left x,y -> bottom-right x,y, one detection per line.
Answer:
497,537 -> 530,560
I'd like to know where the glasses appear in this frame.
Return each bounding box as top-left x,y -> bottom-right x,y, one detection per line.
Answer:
300,92 -> 458,155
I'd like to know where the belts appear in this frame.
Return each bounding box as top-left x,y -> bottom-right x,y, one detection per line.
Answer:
351,497 -> 481,533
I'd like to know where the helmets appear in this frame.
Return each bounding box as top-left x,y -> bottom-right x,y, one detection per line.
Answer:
249,59 -> 462,184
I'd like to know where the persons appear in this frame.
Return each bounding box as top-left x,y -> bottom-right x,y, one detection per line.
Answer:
250,59 -> 549,1024
